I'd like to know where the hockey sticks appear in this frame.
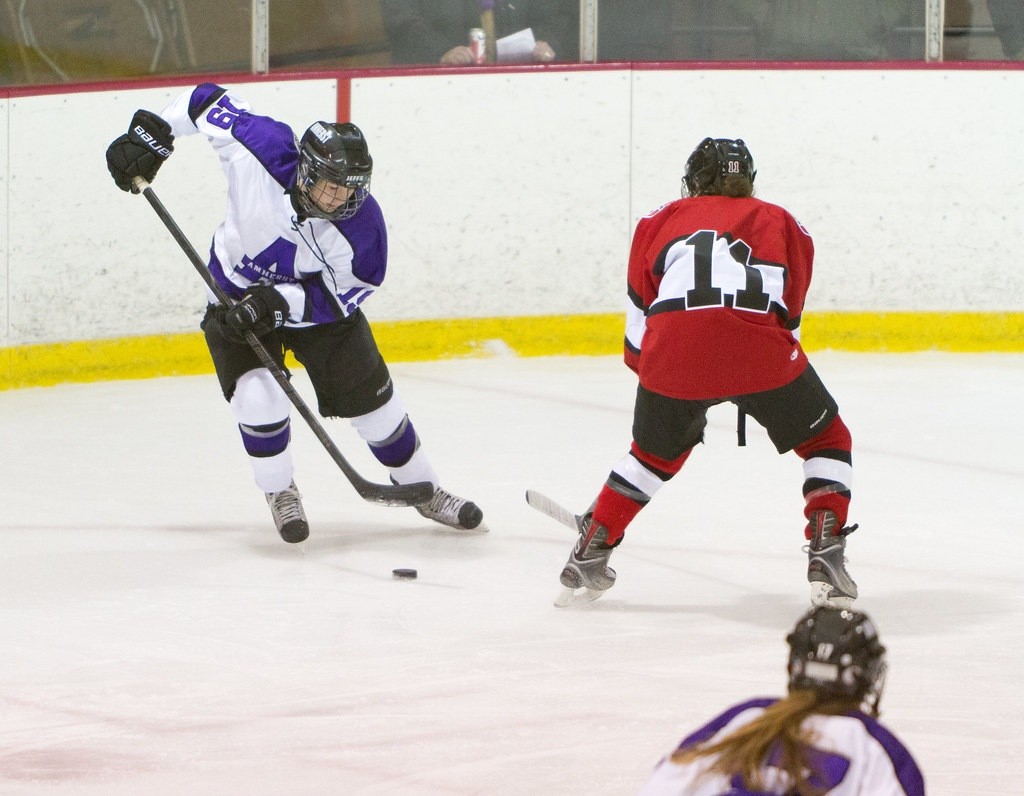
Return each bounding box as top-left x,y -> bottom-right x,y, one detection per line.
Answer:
133,173 -> 435,506
523,485 -> 600,533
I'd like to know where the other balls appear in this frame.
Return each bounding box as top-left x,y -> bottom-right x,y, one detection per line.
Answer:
392,569 -> 418,580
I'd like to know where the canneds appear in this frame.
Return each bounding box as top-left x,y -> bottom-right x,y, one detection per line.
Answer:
470,28 -> 488,68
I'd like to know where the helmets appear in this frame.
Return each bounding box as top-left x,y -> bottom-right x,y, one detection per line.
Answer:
787,607 -> 886,720
681,137 -> 757,198
298,121 -> 373,221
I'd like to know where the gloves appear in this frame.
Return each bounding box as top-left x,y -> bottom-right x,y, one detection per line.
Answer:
209,283 -> 290,345
106,110 -> 175,195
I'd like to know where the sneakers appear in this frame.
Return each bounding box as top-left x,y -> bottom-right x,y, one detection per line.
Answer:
266,477 -> 310,557
802,509 -> 858,607
553,511 -> 624,609
389,473 -> 490,532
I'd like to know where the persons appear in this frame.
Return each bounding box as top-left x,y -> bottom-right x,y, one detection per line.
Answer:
553,136 -> 859,609
373,0 -> 557,64
106,80 -> 491,548
643,602 -> 930,796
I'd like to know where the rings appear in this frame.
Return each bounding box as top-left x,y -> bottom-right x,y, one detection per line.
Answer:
543,52 -> 551,60
